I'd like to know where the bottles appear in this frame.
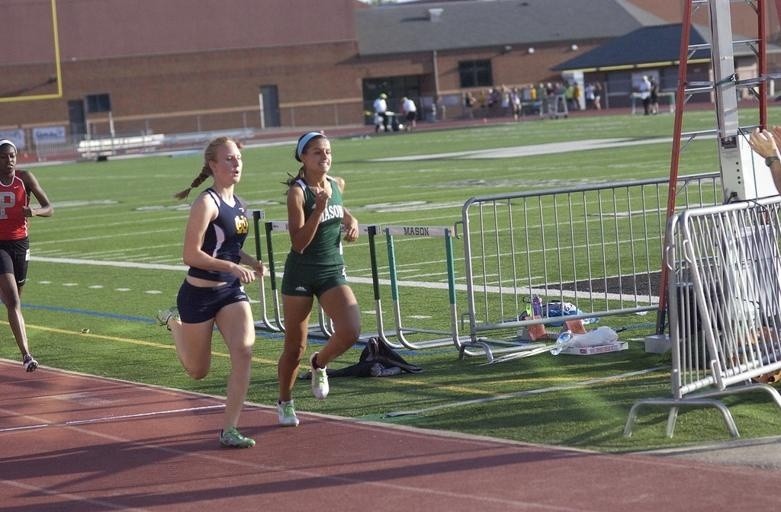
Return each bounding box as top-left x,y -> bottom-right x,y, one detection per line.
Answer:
533,296 -> 543,316
526,296 -> 532,316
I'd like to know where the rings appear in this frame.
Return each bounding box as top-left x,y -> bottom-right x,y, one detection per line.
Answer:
751,142 -> 755,146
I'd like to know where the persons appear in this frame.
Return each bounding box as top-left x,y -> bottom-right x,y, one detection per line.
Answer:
0,138 -> 55,374
639,75 -> 651,116
153,136 -> 269,449
372,92 -> 390,134
464,80 -> 603,122
273,130 -> 365,429
649,74 -> 662,116
401,95 -> 419,134
749,124 -> 781,199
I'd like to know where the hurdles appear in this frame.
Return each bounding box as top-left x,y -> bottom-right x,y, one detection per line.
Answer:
379,223 -> 547,358
264,220 -> 329,336
318,222 -> 418,344
242,209 -> 285,332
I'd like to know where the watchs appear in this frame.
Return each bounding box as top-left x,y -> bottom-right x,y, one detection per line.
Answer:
765,155 -> 780,167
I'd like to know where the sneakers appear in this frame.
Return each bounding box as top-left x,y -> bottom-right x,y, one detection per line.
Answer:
278,399 -> 298,426
219,429 -> 255,448
23,354 -> 38,372
154,306 -> 180,326
309,351 -> 329,400
